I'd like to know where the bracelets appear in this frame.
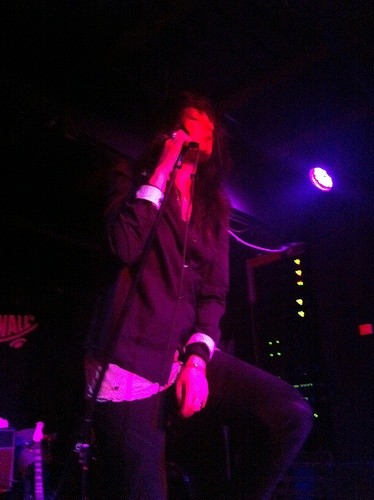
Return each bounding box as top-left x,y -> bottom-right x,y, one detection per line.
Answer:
185,352 -> 208,371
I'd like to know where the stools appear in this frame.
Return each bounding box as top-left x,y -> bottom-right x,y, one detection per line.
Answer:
57,398 -> 233,499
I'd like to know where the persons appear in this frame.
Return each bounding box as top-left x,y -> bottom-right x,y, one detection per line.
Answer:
81,84 -> 316,500
